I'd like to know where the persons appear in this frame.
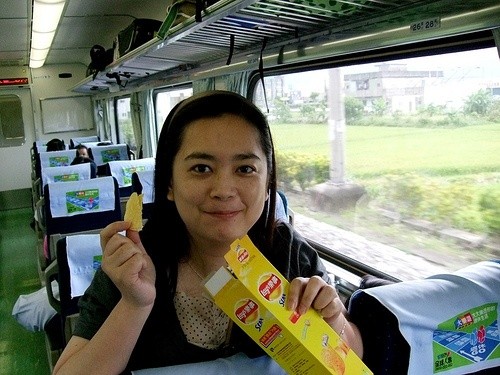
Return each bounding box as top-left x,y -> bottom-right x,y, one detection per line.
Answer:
47,89 -> 365,375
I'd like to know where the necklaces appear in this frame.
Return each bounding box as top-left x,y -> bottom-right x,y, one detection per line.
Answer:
184,257 -> 208,282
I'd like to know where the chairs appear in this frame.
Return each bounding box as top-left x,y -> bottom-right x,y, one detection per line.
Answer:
29,135 -> 500,375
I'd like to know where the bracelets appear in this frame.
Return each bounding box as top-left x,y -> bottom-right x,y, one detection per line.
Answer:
338,318 -> 350,339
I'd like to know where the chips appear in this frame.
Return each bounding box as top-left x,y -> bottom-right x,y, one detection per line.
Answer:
199,234 -> 375,375
124,192 -> 144,232
